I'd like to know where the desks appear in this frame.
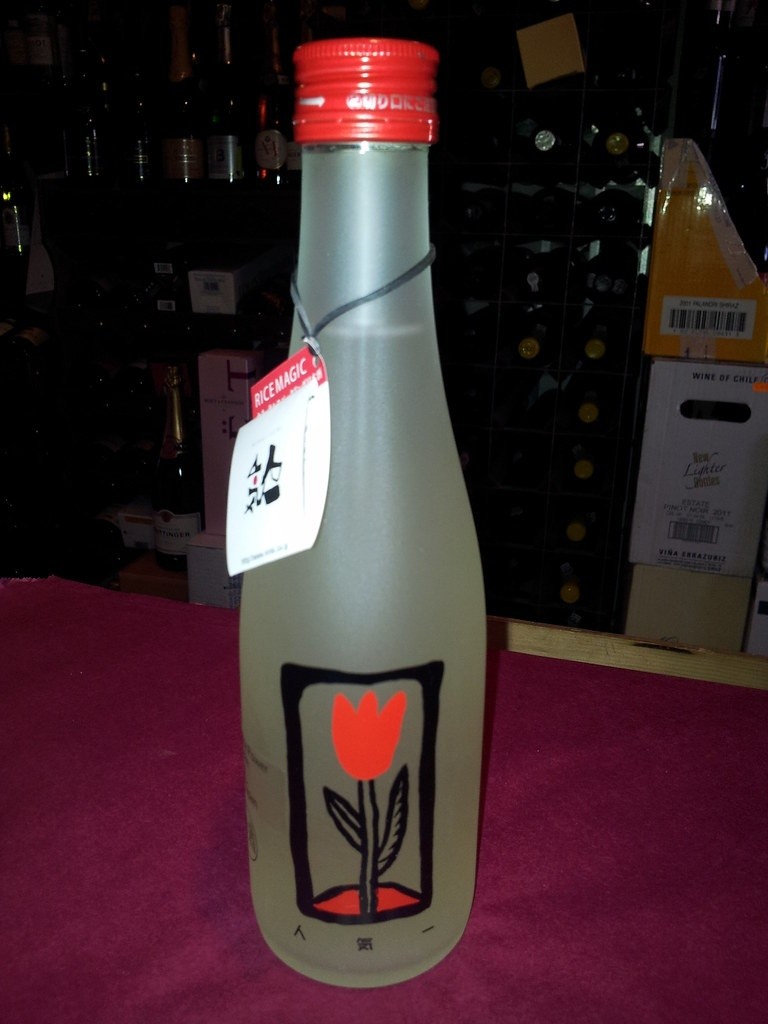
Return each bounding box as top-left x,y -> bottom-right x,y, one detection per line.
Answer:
0,576 -> 768,1024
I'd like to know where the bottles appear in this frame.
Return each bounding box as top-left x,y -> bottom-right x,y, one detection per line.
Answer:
239,38 -> 488,989
1,0 -> 635,635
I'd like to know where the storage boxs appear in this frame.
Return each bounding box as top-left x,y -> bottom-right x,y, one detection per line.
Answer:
626,565 -> 752,650
629,356 -> 768,577
189,249 -> 291,314
186,532 -> 242,608
198,350 -> 263,535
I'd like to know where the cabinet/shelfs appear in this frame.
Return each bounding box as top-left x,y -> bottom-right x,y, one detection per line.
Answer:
0,0 -> 688,634
